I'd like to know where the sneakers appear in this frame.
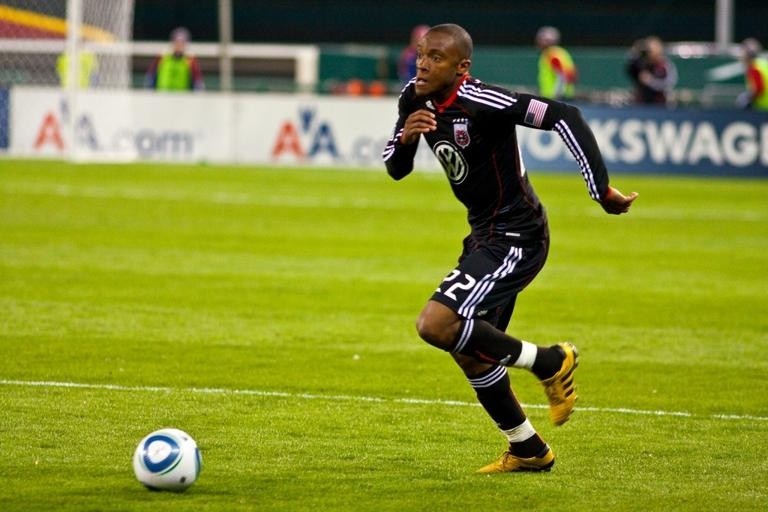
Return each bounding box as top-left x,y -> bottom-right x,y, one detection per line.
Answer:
475,442 -> 555,474
535,342 -> 580,427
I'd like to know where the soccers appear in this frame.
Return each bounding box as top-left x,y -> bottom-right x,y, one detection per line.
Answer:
133,428 -> 203,491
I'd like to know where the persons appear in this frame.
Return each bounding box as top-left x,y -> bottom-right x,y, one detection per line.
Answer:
143,27 -> 204,92
737,39 -> 768,113
534,28 -> 580,99
380,22 -> 640,476
56,37 -> 100,86
624,36 -> 678,108
398,27 -> 431,83
